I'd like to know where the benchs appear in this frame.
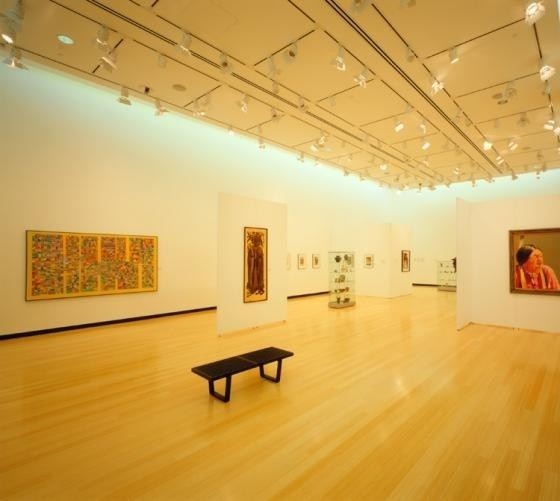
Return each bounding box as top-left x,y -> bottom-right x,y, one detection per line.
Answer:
191,345 -> 294,403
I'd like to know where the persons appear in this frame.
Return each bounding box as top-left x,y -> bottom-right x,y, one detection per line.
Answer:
246,231 -> 265,296
515,244 -> 560,290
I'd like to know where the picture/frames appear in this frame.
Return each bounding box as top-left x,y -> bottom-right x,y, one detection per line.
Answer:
242,226 -> 270,303
363,253 -> 374,268
401,249 -> 411,272
25,228 -> 161,301
508,228 -> 560,295
296,252 -> 322,270
